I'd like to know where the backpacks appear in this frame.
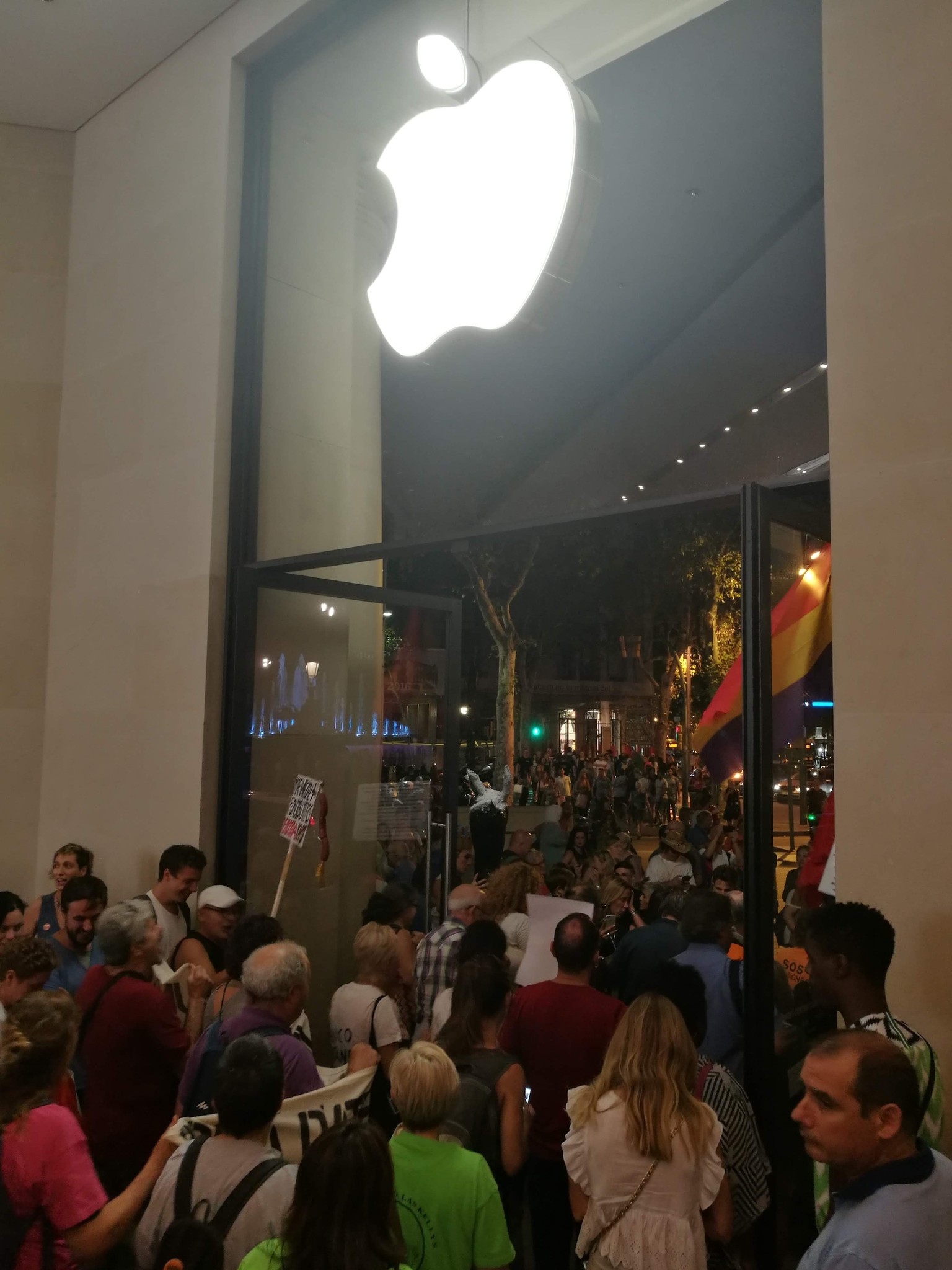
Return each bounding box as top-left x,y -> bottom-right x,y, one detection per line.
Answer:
0,1091 -> 55,1269
152,1135 -> 289,1270
439,1054 -> 507,1172
179,1019 -> 287,1116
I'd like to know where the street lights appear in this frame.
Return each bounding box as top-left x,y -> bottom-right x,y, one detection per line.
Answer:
305,660 -> 319,700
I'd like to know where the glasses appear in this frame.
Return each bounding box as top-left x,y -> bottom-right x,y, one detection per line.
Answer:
409,903 -> 416,910
465,904 -> 490,918
614,872 -> 631,878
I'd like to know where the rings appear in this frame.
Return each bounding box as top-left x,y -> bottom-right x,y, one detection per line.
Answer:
607,932 -> 609,936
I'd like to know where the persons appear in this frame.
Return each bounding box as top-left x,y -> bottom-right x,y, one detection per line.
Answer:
0,744 -> 952,1269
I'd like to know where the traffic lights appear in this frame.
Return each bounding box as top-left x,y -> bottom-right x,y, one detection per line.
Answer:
532,727 -> 540,735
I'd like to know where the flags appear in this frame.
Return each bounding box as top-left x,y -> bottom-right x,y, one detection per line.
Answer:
690,544 -> 833,782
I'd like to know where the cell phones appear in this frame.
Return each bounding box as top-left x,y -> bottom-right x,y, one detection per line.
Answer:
606,914 -> 616,932
682,875 -> 690,881
522,1085 -> 531,1109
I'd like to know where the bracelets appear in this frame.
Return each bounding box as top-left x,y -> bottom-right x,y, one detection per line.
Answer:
630,909 -> 639,917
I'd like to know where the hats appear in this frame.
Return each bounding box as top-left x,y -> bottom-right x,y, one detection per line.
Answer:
197,885 -> 242,911
660,821 -> 692,853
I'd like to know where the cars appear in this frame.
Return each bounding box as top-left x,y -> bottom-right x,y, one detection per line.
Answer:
774,768 -> 834,805
736,763 -> 815,796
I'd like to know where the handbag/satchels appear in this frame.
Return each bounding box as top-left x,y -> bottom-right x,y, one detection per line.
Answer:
574,794 -> 588,808
58,1063 -> 86,1126
554,790 -> 566,804
355,995 -> 401,1135
667,755 -> 674,764
